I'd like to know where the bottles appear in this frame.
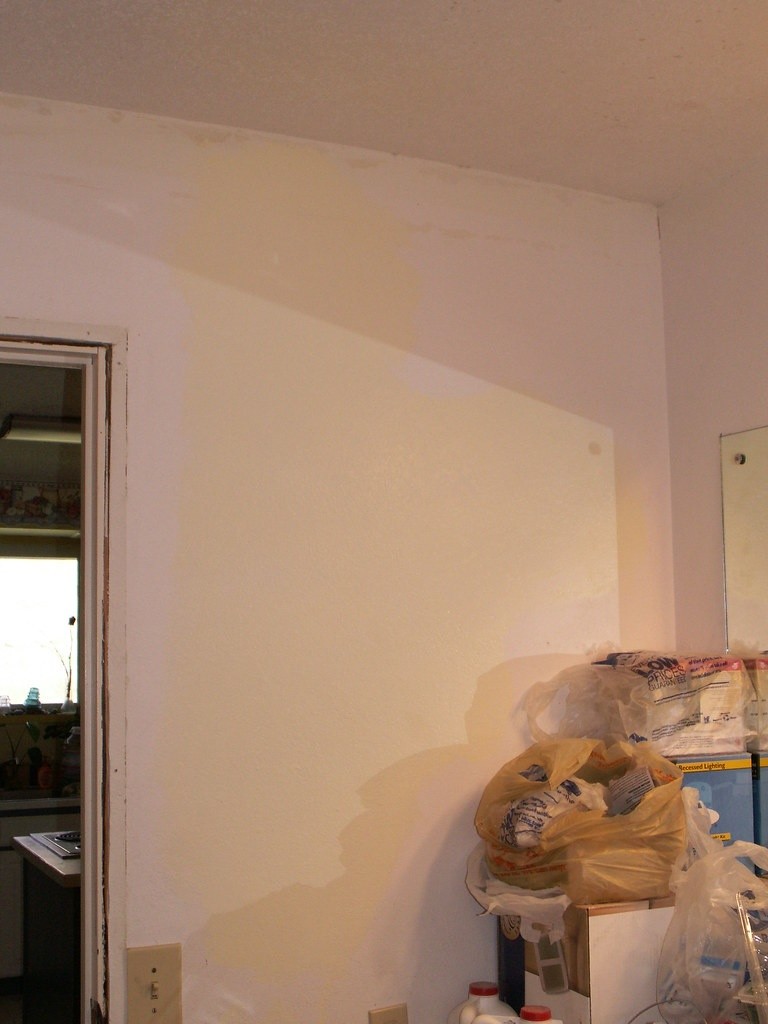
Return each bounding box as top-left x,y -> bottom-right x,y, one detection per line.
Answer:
512,1006 -> 562,1024
447,982 -> 518,1024
62,727 -> 81,798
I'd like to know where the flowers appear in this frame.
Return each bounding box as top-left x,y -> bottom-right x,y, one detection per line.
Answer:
56,617 -> 76,696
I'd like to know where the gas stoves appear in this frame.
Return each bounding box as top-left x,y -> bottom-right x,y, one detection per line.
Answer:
31,830 -> 82,859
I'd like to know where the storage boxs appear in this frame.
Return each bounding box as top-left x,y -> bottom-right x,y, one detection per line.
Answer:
664,753 -> 754,875
749,751 -> 768,877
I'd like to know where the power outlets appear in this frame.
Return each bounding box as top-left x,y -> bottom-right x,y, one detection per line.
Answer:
369,1003 -> 408,1024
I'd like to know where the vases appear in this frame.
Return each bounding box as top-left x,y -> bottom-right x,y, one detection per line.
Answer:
59,685 -> 76,714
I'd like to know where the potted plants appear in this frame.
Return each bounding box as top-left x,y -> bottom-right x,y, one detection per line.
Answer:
0,720 -> 42,786
